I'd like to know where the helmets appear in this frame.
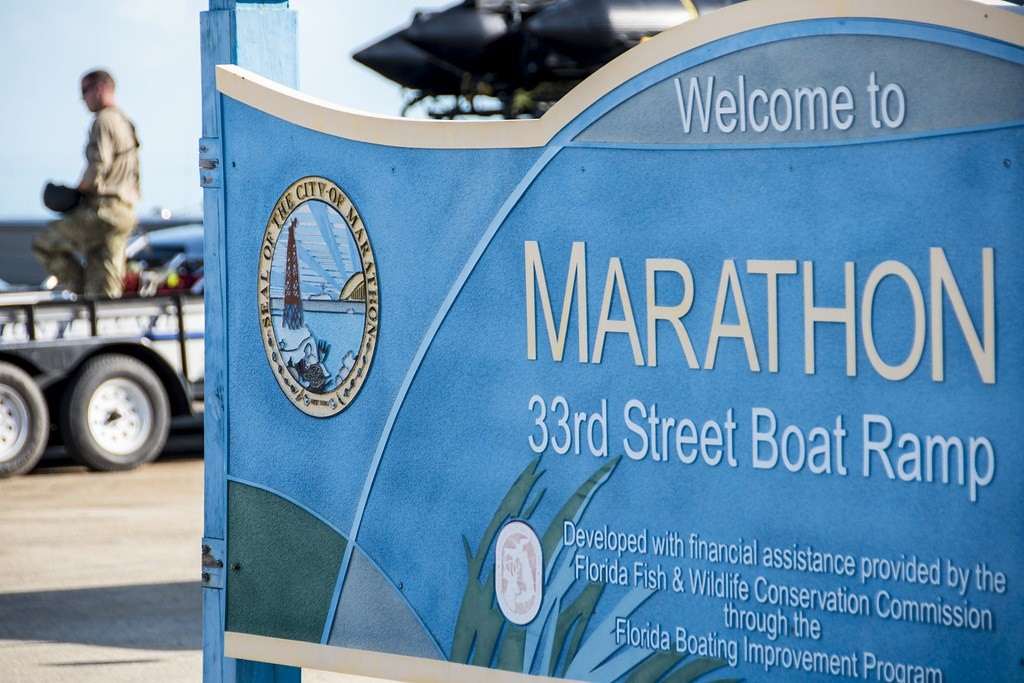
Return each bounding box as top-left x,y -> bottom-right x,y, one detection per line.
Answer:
43,183 -> 81,211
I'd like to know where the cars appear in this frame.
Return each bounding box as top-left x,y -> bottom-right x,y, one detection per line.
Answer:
0,225 -> 204,306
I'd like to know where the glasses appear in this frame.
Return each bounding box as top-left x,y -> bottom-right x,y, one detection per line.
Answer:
81,83 -> 95,94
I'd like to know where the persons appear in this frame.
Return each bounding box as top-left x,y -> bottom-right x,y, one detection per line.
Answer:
30,70 -> 142,301
